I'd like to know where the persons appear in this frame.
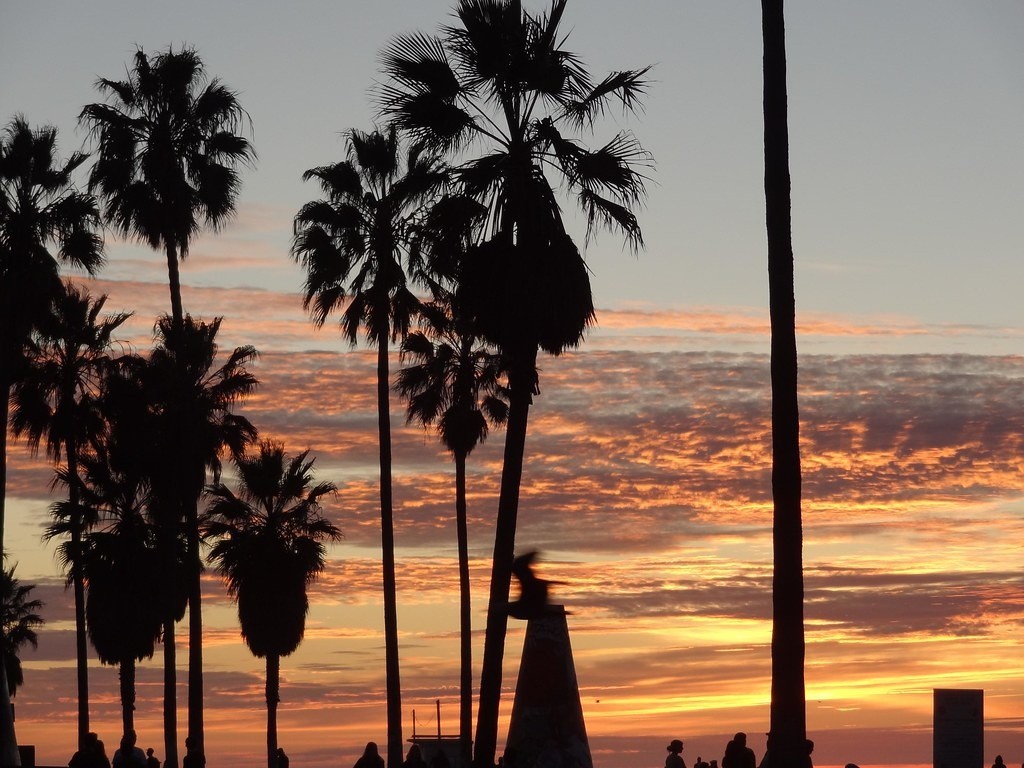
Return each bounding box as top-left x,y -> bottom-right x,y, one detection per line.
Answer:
403,744 -> 428,768
664,740 -> 688,768
353,742 -> 385,768
694,732 -> 757,768
806,739 -> 814,768
183,738 -> 206,768
991,755 -> 1007,768
146,748 -> 160,768
68,732 -> 112,768
112,730 -> 148,768
277,748 -> 289,768
